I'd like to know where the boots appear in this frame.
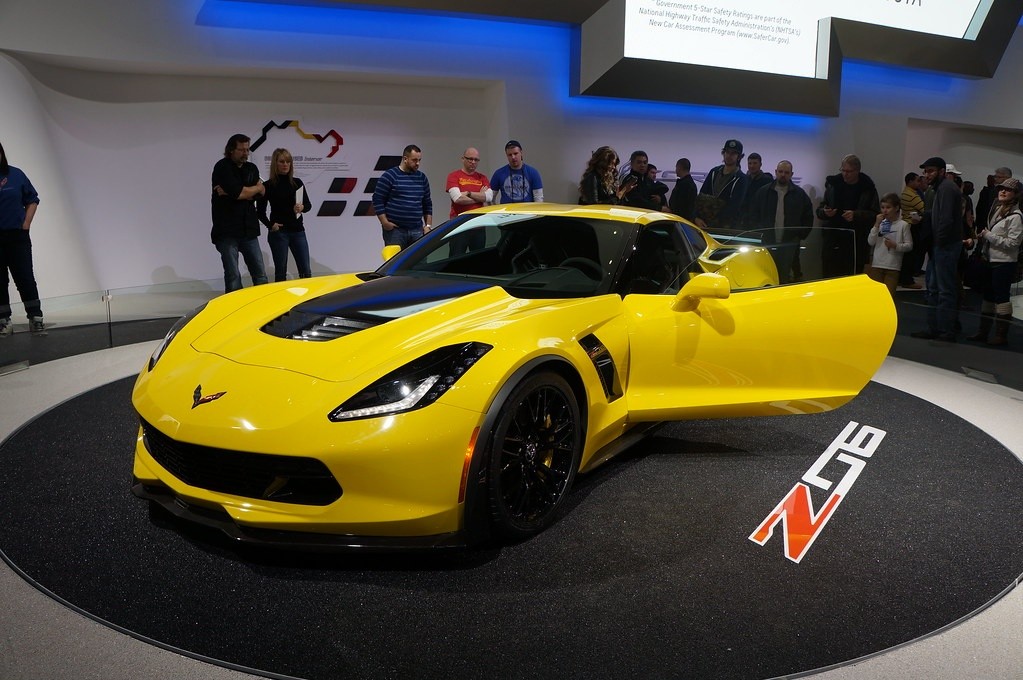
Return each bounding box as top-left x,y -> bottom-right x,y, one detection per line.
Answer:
985,302 -> 1013,349
966,301 -> 994,342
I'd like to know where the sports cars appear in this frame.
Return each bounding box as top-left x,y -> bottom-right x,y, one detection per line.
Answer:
130,200 -> 900,551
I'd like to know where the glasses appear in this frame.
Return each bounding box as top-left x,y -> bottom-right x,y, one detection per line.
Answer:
464,156 -> 480,162
922,168 -> 938,175
839,167 -> 858,173
233,148 -> 250,153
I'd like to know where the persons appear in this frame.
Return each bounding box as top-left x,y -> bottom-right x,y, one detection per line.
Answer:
256,148 -> 313,283
0,142 -> 45,336
445,147 -> 493,258
490,140 -> 544,203
211,133 -> 268,294
371,145 -> 433,255
578,139 -> 1022,342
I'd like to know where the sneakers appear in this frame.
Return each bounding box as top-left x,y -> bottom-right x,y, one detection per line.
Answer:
29,316 -> 48,336
0,318 -> 13,338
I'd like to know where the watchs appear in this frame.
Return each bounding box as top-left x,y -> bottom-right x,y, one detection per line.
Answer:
466,191 -> 471,198
425,224 -> 433,231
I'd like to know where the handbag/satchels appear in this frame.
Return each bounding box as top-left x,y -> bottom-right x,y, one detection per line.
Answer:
695,192 -> 734,230
982,239 -> 990,263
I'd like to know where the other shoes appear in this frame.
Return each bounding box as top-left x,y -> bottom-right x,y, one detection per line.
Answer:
902,283 -> 922,289
935,320 -> 962,343
910,324 -> 937,339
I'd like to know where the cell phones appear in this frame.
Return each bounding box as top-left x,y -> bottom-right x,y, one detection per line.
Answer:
910,212 -> 921,222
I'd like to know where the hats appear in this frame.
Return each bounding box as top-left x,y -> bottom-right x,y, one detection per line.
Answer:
722,140 -> 743,154
995,178 -> 1023,191
945,164 -> 962,175
505,140 -> 522,150
919,157 -> 946,171
